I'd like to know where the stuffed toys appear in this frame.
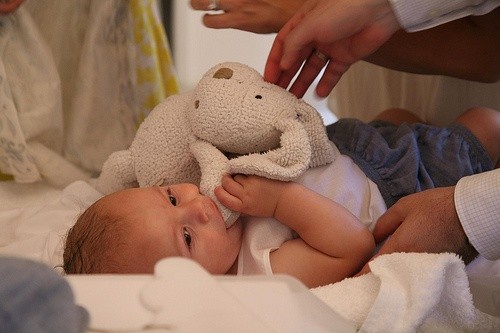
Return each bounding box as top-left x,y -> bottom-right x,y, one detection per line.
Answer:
96,60 -> 336,229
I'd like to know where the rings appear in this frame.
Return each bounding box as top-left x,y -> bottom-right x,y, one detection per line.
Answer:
209,0 -> 220,11
315,49 -> 330,62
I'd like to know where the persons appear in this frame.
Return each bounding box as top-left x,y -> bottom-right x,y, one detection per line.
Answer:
62,107 -> 500,289
264,0 -> 500,278
